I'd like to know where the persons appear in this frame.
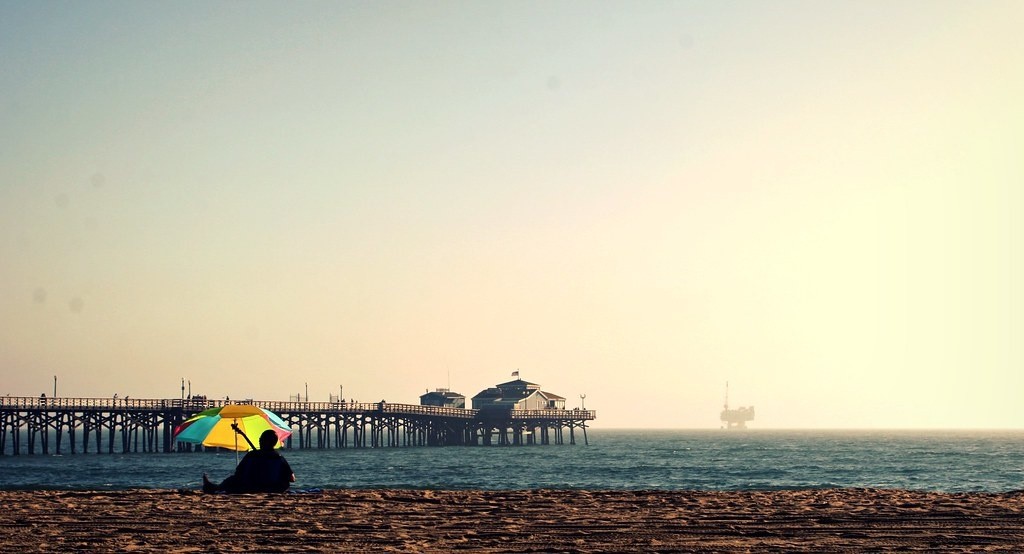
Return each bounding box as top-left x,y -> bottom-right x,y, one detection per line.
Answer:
125,396 -> 129,406
202,430 -> 296,493
224,396 -> 229,404
113,393 -> 118,406
38,393 -> 46,408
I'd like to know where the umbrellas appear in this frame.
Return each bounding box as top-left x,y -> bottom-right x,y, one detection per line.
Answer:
174,403 -> 294,466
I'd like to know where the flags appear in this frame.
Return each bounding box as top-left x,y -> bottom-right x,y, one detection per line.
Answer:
511,371 -> 518,376
